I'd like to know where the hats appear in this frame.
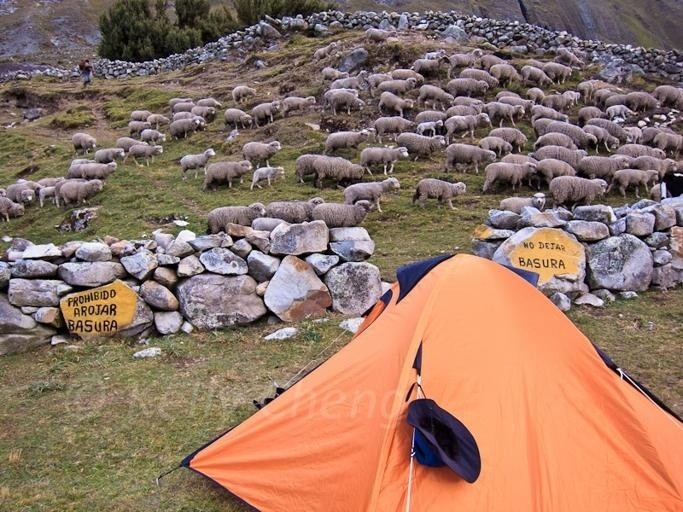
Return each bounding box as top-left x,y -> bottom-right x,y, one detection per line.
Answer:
408,397 -> 481,483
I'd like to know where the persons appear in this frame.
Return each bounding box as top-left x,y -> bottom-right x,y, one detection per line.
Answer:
81,59 -> 92,83
78,58 -> 84,69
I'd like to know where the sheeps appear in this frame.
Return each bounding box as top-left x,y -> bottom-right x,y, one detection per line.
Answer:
1,85 -> 288,236
268,26 -> 683,226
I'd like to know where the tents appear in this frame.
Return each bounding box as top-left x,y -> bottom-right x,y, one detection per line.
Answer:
150,248 -> 682,512
